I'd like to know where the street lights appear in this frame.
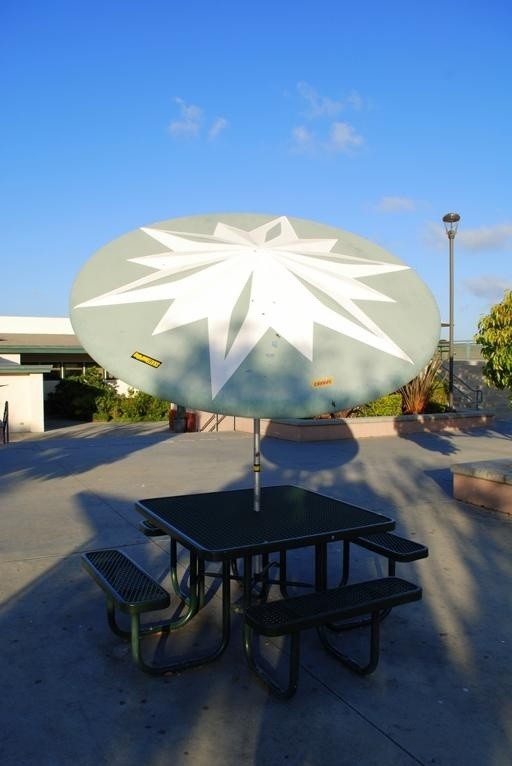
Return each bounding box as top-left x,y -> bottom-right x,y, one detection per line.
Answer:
443,213 -> 460,413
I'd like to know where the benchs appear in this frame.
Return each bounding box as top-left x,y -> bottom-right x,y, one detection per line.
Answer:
245,576 -> 422,700
340,532 -> 429,586
83,549 -> 227,676
139,520 -> 167,537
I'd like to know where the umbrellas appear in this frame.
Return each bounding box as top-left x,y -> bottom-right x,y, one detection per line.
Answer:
65,205 -> 445,519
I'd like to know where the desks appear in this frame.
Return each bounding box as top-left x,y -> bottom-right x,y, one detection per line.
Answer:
128,484 -> 394,662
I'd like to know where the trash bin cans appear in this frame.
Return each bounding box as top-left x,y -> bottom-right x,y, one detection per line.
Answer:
173,417 -> 186,433
